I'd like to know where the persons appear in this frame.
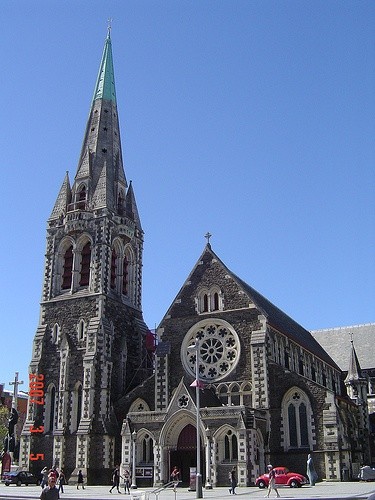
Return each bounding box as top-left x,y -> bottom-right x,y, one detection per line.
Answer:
172,467 -> 179,492
229,465 -> 238,495
123,469 -> 131,495
41,466 -> 65,493
263,465 -> 280,498
77,470 -> 85,489
109,469 -> 121,493
39,477 -> 59,500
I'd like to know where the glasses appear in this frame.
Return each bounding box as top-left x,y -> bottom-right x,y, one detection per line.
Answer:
50,481 -> 56,483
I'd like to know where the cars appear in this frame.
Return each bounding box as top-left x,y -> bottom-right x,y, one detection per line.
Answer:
255,466 -> 308,488
1,470 -> 41,486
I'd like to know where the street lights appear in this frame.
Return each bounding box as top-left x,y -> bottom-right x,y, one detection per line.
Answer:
131,430 -> 139,489
205,425 -> 213,489
186,337 -> 204,498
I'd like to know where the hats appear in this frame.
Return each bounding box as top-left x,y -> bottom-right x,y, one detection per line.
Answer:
267,464 -> 272,468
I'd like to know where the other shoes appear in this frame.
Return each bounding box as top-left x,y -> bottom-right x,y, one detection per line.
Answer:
275,495 -> 280,498
83,488 -> 85,489
62,492 -> 64,493
118,491 -> 121,493
233,493 -> 237,495
264,495 -> 269,498
77,487 -> 78,489
109,490 -> 112,493
229,489 -> 231,494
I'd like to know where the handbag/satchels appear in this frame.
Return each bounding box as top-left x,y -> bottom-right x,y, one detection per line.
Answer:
59,476 -> 63,480
